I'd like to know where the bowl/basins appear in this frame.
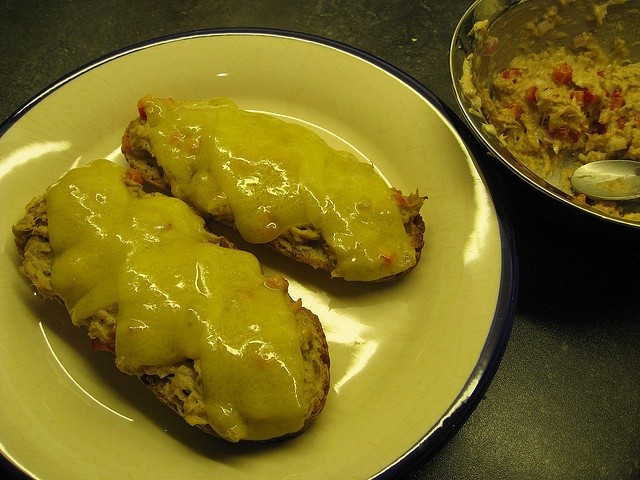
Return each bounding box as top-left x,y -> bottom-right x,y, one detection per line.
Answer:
449,0 -> 640,229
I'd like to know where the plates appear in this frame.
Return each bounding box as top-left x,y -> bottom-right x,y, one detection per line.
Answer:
0,30 -> 518,480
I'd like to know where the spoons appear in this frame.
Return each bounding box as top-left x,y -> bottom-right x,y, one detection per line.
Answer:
570,159 -> 640,203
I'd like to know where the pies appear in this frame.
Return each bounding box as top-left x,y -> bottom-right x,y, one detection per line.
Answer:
14,159 -> 329,445
120,92 -> 428,286
